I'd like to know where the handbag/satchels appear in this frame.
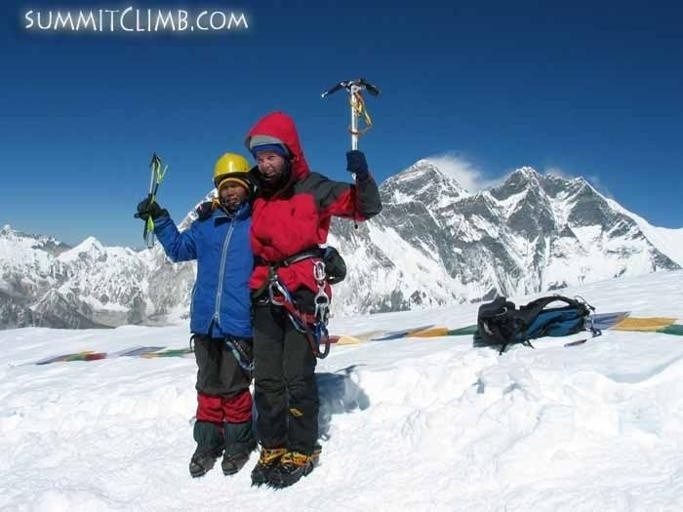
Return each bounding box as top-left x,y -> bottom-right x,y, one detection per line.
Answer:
477,296 -> 588,345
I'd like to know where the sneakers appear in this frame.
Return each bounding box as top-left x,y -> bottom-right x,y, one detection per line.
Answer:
189,450 -> 215,476
252,447 -> 311,487
222,449 -> 248,474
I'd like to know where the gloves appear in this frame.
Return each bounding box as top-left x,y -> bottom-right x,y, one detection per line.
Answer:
347,149 -> 367,173
132,198 -> 162,220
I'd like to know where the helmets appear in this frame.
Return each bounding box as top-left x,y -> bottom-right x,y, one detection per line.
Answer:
215,154 -> 251,191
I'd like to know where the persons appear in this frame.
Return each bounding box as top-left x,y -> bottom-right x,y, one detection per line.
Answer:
196,111 -> 383,489
133,152 -> 256,478
